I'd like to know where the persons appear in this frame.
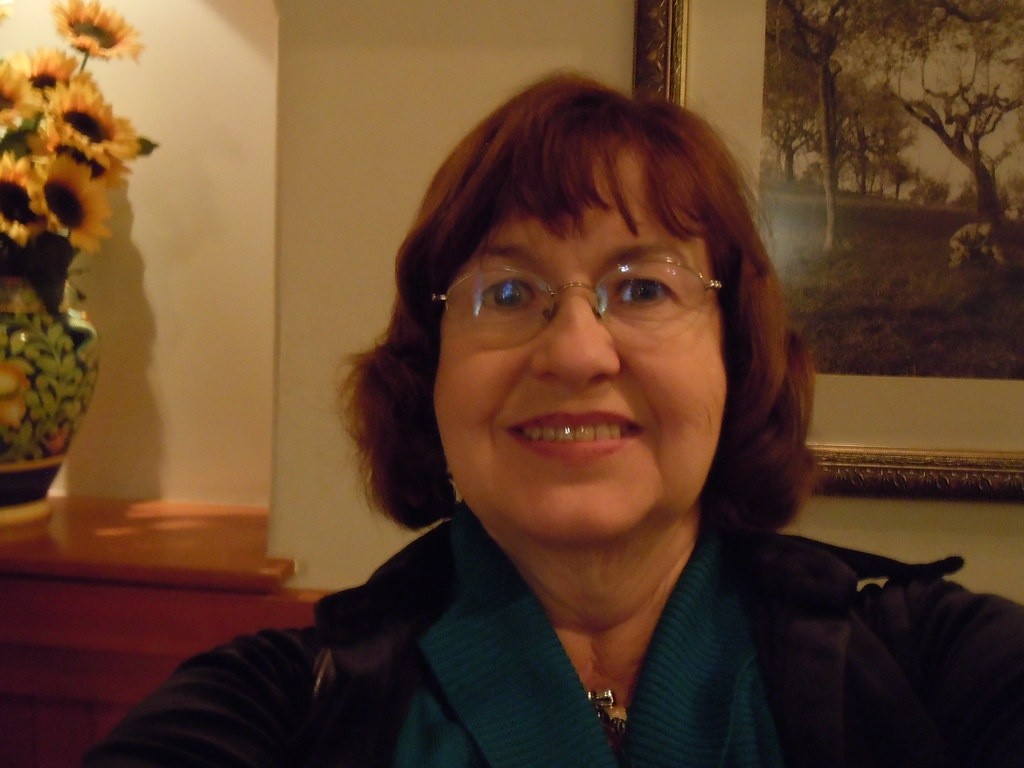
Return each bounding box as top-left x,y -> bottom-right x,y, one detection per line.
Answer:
86,75 -> 1024,768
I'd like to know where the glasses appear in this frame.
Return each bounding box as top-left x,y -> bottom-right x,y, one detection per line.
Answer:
431,258 -> 722,350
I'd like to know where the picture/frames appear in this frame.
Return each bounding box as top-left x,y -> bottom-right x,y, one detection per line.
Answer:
630,0 -> 1024,500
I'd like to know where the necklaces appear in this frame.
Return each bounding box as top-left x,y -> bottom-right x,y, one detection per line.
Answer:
588,688 -> 626,738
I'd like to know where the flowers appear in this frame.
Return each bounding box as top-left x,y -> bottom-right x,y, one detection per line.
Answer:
0,0 -> 158,301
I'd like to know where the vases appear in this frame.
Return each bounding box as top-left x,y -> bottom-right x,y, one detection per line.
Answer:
0,276 -> 99,509
